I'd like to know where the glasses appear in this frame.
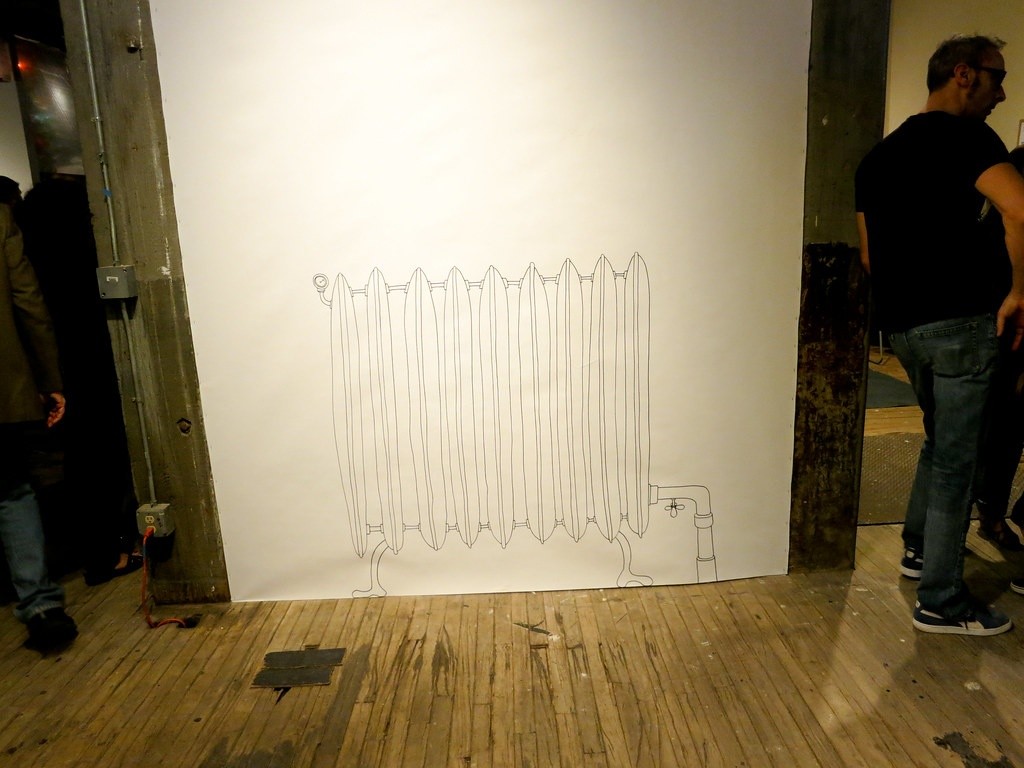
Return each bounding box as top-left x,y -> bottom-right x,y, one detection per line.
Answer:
950,62 -> 1007,84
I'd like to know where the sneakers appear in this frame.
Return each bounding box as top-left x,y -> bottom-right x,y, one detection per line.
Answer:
912,597 -> 1012,634
900,546 -> 924,581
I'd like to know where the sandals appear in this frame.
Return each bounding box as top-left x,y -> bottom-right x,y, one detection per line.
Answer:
85,554 -> 143,586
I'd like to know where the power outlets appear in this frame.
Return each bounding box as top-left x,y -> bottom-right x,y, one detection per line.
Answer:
136,504 -> 175,538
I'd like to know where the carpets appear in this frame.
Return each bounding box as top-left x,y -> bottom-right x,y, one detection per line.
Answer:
865,369 -> 920,409
857,432 -> 1024,526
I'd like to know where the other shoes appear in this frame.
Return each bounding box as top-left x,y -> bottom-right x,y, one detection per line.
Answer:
1010,576 -> 1024,595
30,608 -> 76,653
977,519 -> 1020,551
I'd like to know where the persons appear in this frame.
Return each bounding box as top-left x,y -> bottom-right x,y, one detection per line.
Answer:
16,121 -> 149,586
0,173 -> 78,654
994,138 -> 1024,599
853,32 -> 1016,638
